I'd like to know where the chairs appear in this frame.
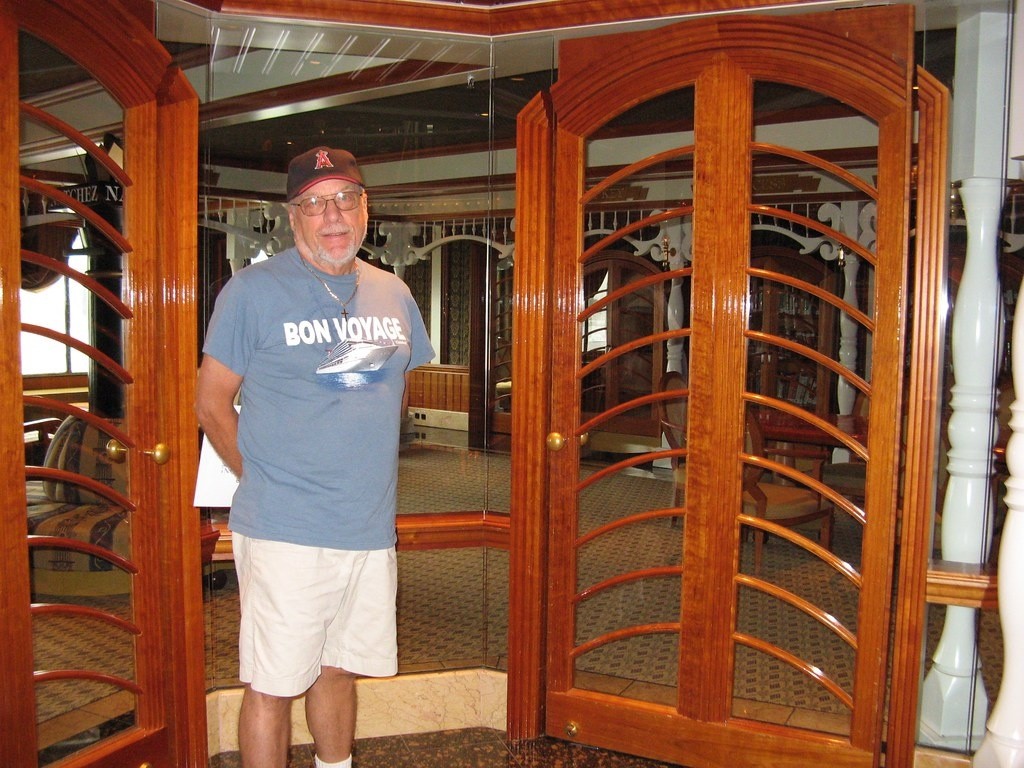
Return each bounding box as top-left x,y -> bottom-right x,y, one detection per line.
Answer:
658,371 -> 688,526
743,406 -> 835,577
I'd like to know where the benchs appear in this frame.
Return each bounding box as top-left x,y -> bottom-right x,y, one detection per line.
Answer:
24,414 -> 238,598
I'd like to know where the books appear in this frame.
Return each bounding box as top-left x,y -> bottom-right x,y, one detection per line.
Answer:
750,285 -> 819,405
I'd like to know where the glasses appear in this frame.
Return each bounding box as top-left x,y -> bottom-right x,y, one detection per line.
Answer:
289,191 -> 364,217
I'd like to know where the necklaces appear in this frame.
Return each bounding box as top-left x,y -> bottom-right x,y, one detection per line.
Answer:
296,246 -> 360,322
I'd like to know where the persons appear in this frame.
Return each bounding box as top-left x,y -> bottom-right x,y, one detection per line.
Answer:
193,146 -> 436,768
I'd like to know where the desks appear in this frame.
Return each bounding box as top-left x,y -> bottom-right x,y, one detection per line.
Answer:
750,409 -> 870,449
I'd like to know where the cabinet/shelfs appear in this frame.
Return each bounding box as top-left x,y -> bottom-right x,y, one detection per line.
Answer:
486,233 -> 1013,456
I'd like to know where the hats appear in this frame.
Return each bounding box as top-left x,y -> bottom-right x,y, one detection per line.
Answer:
284,146 -> 365,203
